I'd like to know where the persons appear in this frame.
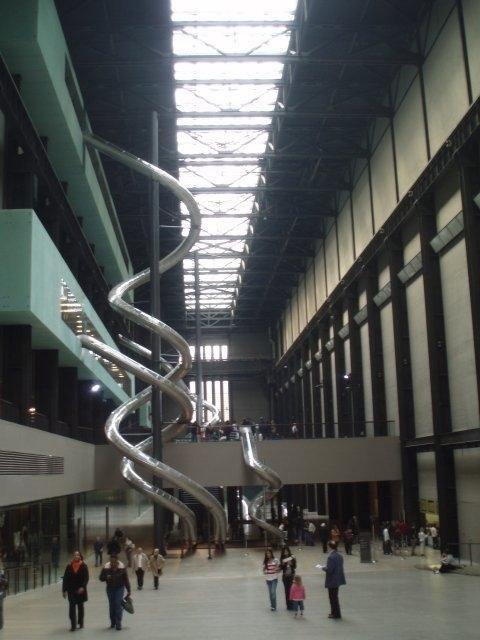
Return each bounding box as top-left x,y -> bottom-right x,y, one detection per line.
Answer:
319,540 -> 346,618
16,525 -> 28,566
280,546 -> 296,610
277,503 -> 360,556
289,575 -> 305,617
262,548 -> 282,610
434,549 -> 459,574
50,527 -> 169,630
0,565 -> 8,629
288,415 -> 301,439
188,415 -> 279,443
379,516 -> 441,556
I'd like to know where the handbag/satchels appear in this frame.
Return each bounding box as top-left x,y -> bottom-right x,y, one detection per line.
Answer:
123,595 -> 134,614
158,568 -> 162,574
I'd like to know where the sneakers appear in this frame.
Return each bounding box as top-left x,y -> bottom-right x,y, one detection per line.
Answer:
70,623 -> 121,631
